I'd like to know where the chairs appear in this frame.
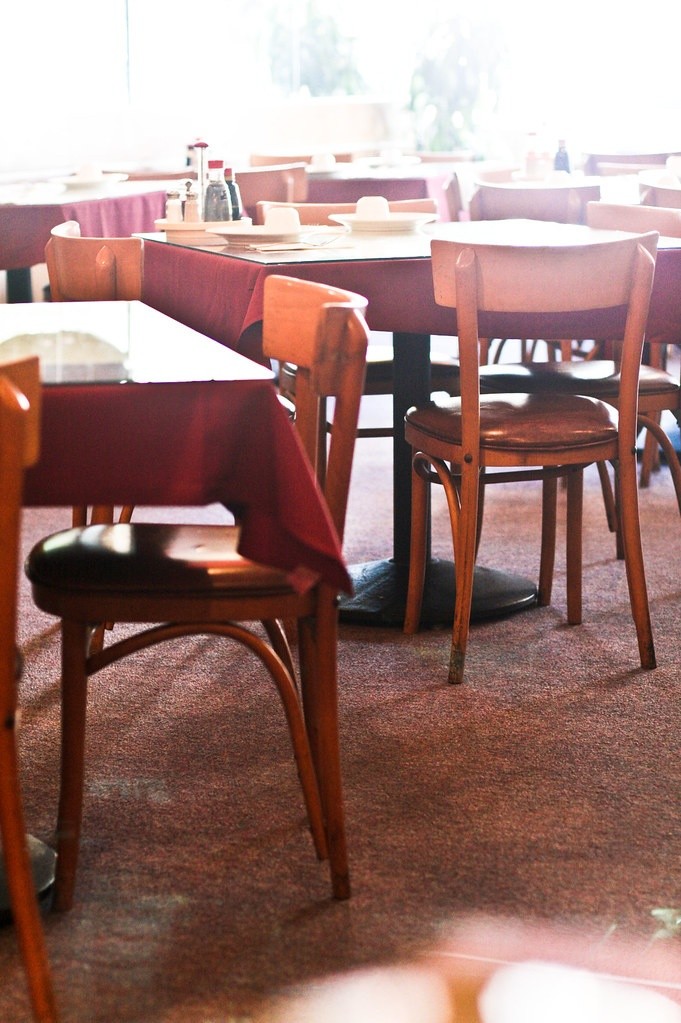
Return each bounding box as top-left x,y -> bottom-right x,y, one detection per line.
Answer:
449,202 -> 680,623
403,232 -> 661,684
1,356 -> 58,1023
467,181 -> 603,365
42,219 -> 145,656
24,272 -> 371,915
253,198 -> 439,225
232,163 -> 307,207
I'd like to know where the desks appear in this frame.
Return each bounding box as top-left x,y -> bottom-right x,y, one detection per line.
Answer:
0,146 -> 681,928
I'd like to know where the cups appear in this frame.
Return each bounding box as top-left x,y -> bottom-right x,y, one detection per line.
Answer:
265,208 -> 300,228
666,155 -> 681,173
356,196 -> 389,215
573,169 -> 585,186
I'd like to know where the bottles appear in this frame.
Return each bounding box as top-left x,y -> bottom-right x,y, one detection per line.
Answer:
165,191 -> 183,223
186,144 -> 196,171
554,139 -> 569,172
185,191 -> 203,222
204,160 -> 231,222
224,168 -> 242,220
527,133 -> 551,173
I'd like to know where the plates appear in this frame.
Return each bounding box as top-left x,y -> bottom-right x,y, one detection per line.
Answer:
51,173 -> 129,189
205,227 -> 304,253
328,213 -> 439,231
